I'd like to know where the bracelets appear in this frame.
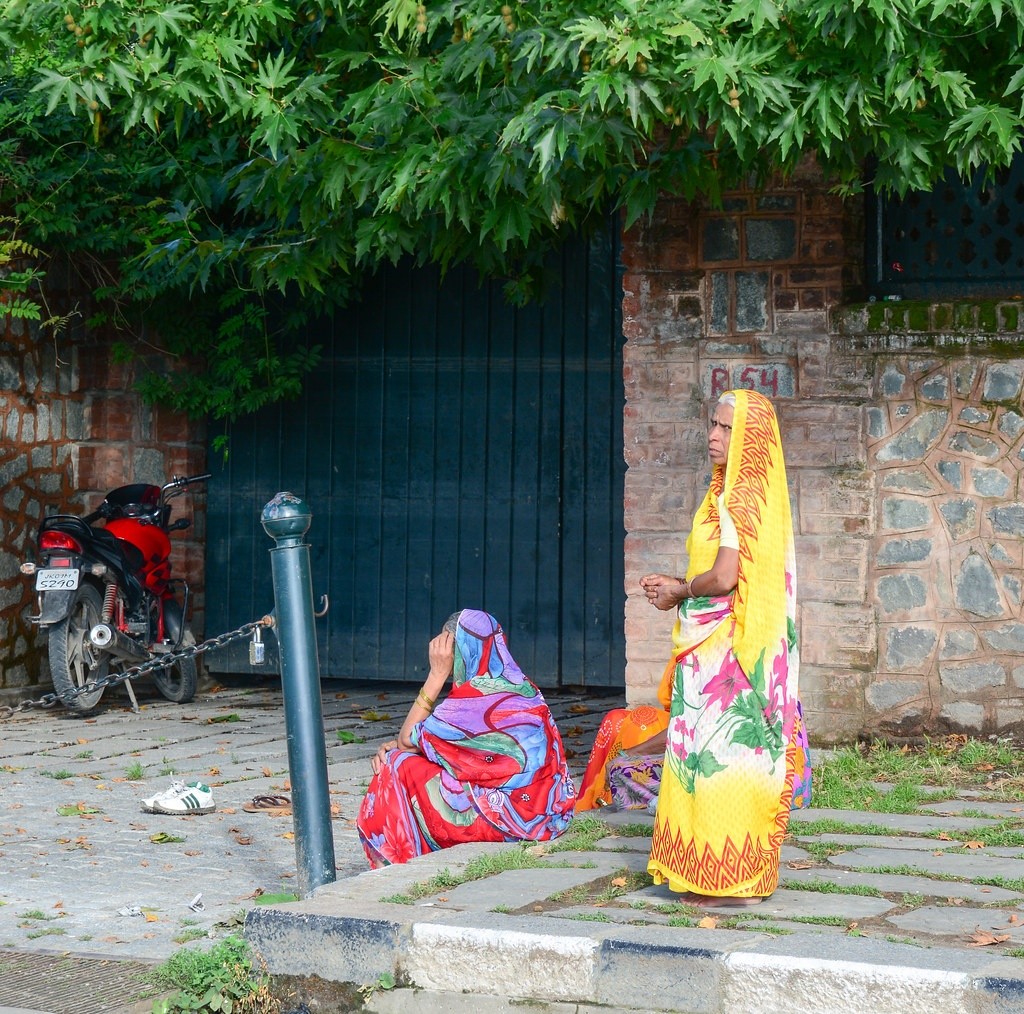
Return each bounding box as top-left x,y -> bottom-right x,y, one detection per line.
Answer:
616,750 -> 628,758
675,577 -> 687,585
686,576 -> 699,599
415,686 -> 434,713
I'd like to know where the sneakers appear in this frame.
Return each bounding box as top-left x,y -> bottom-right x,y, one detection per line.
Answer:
140,780 -> 186,812
154,783 -> 215,814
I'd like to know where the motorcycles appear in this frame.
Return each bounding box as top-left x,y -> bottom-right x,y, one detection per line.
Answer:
19,472 -> 215,715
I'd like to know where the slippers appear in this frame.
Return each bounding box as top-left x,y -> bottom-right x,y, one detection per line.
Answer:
242,794 -> 292,814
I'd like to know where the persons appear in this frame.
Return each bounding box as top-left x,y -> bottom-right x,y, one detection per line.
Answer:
356,610 -> 577,870
639,388 -> 799,908
575,655 -> 811,817
